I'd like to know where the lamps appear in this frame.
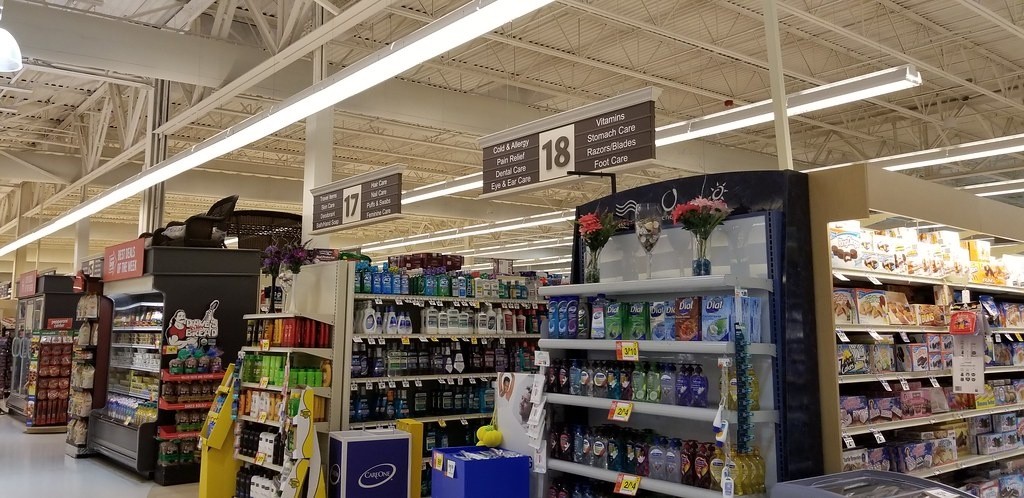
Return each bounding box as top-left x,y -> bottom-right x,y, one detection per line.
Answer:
0,0 -> 22,73
0,0 -> 1024,265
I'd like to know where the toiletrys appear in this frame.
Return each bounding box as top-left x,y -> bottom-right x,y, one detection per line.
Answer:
353,258 -> 769,498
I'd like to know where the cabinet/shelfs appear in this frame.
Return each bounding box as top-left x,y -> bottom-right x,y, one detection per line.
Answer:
4,163 -> 824,498
808,162 -> 1024,497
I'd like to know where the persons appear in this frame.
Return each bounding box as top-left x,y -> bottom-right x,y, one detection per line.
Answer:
518,386 -> 533,422
499,372 -> 515,401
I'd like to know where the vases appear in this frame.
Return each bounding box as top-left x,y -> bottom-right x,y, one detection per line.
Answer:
690,230 -> 712,276
634,203 -> 661,278
583,251 -> 601,284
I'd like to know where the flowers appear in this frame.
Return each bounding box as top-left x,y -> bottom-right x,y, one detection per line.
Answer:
637,216 -> 661,251
567,213 -> 613,252
670,194 -> 734,230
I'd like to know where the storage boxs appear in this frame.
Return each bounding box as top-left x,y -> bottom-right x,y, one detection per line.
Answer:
829,228 -> 1024,498
327,427 -> 411,498
396,418 -> 423,498
430,446 -> 533,498
